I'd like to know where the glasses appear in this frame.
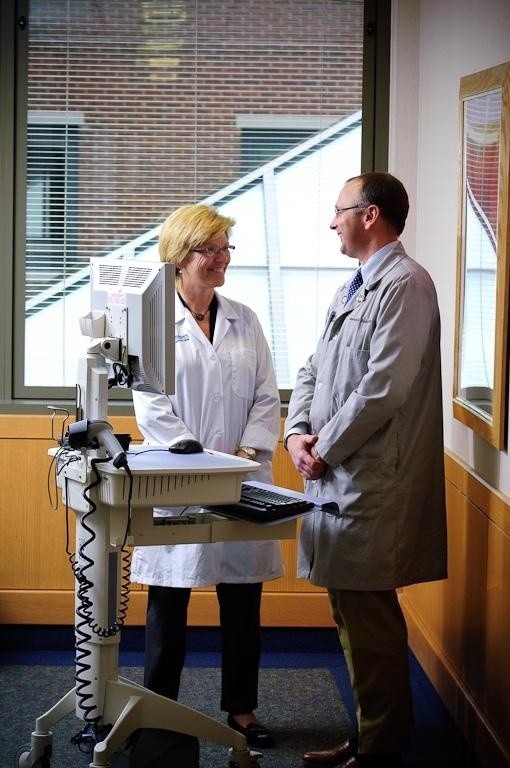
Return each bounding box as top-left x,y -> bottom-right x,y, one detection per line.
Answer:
194,244 -> 235,258
333,203 -> 359,216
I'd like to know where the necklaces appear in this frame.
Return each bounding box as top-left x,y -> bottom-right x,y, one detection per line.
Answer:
190,309 -> 210,322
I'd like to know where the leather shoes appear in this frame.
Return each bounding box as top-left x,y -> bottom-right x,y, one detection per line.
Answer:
227,713 -> 273,749
331,754 -> 363,768
303,738 -> 354,765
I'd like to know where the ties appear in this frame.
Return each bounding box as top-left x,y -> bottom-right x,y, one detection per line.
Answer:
344,270 -> 363,308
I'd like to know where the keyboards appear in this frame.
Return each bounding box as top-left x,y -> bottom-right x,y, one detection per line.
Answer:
211,481 -> 315,522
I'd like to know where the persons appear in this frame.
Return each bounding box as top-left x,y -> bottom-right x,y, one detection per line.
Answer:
277,170 -> 451,768
130,203 -> 286,752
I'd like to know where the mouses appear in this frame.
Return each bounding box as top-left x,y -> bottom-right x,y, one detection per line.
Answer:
169,440 -> 203,454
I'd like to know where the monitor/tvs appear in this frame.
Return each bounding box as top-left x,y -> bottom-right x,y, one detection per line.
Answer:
89,256 -> 175,395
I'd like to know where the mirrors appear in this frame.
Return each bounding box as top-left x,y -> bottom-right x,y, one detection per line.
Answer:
451,63 -> 510,451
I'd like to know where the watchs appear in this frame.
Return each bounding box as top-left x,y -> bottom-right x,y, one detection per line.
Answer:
238,447 -> 257,461
